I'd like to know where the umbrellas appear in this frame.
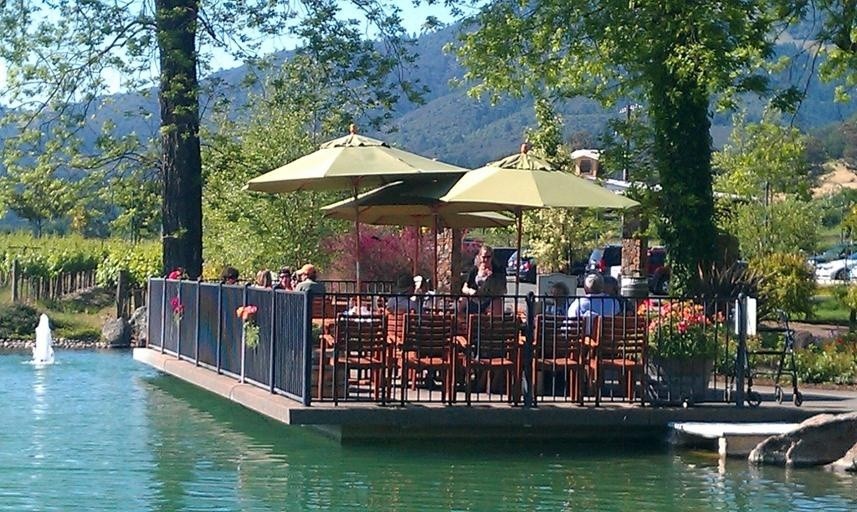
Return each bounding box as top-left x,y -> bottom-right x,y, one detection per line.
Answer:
321,210 -> 515,279
317,179 -> 516,278
394,144 -> 643,318
240,122 -> 468,307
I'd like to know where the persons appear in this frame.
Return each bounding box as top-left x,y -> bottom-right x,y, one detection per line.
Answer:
603,275 -> 636,316
560,270 -> 621,339
460,245 -> 500,314
251,270 -> 273,291
544,282 -> 570,316
385,274 -> 426,315
409,278 -> 440,307
168,267 -> 182,280
221,265 -> 238,287
290,272 -> 297,289
293,262 -> 323,295
272,268 -> 292,291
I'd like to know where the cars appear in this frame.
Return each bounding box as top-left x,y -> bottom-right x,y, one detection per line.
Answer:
799,240 -> 857,287
457,236 -> 672,298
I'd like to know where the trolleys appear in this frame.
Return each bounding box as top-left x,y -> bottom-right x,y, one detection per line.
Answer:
724,303 -> 805,409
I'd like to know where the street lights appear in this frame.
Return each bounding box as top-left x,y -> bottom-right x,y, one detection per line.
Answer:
757,179 -> 769,245
618,102 -> 643,239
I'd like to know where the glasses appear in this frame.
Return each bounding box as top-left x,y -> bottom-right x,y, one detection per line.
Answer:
481,255 -> 491,259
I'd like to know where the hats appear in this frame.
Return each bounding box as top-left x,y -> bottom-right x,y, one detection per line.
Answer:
279,269 -> 291,278
583,271 -> 605,290
295,264 -> 316,276
396,274 -> 414,292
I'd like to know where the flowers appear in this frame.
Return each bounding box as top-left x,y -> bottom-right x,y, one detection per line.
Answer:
637,298 -> 724,354
236,303 -> 260,350
168,296 -> 184,328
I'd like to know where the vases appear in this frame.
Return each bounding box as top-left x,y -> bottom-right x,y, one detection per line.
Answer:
654,355 -> 713,403
